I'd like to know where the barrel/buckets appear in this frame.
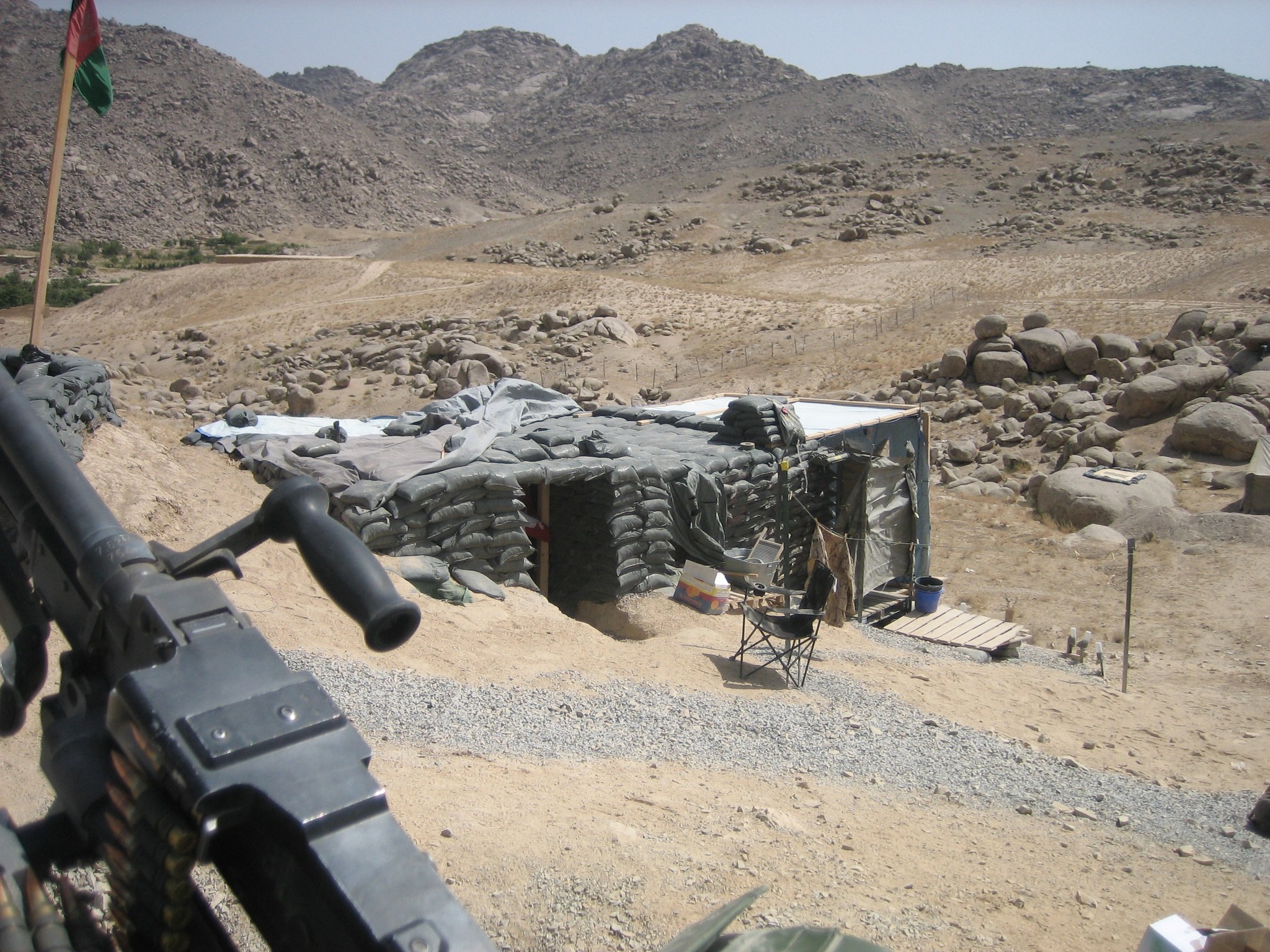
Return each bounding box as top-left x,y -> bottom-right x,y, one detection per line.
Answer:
914,575 -> 944,592
723,547 -> 780,590
915,586 -> 945,613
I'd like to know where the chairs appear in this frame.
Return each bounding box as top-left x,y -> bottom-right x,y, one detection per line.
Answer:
730,559 -> 835,689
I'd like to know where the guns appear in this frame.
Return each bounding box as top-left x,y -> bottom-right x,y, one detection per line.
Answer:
0,336 -> 492,952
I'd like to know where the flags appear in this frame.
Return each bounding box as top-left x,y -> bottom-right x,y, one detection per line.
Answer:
60,0 -> 113,117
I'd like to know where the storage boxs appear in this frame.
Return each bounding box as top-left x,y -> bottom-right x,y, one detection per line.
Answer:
673,559 -> 731,615
1136,902 -> 1270,952
